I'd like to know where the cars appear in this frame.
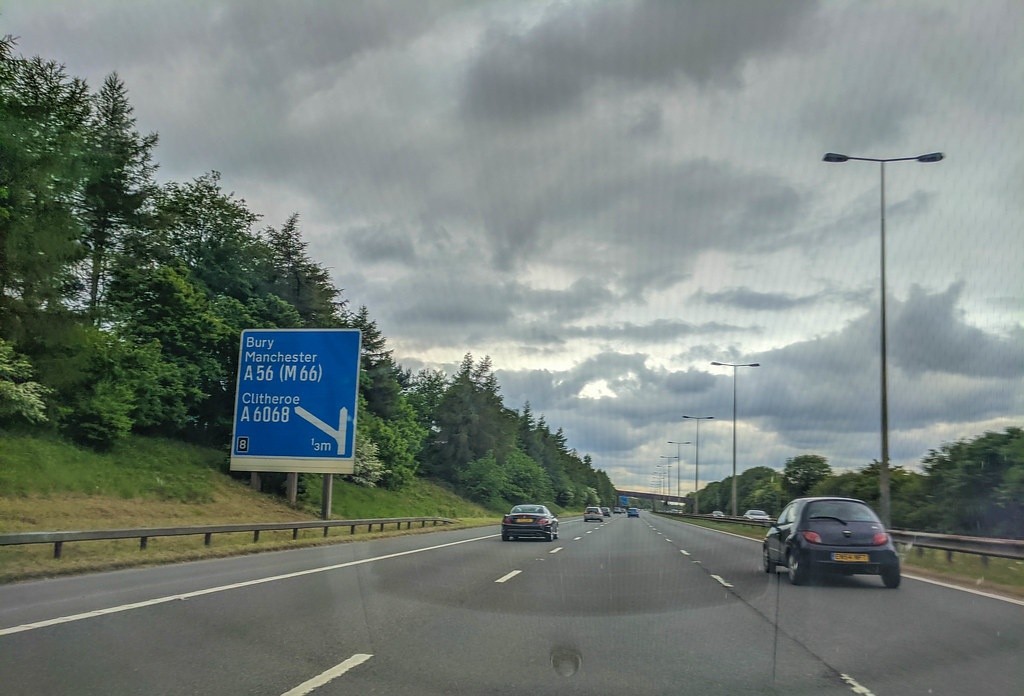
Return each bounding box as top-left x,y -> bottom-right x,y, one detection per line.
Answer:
601,507 -> 611,517
501,505 -> 559,542
710,511 -> 724,521
584,507 -> 604,522
742,510 -> 769,527
762,497 -> 900,588
628,508 -> 639,518
613,507 -> 626,514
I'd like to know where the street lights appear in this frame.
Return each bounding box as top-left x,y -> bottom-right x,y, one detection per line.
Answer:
650,456 -> 678,501
823,153 -> 943,529
711,361 -> 760,518
683,416 -> 715,514
667,441 -> 690,513
